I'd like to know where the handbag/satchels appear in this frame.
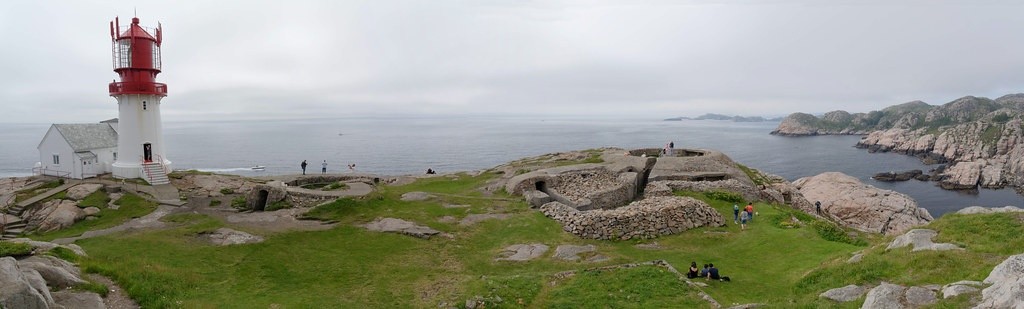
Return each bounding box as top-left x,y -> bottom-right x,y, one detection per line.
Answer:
687,272 -> 692,278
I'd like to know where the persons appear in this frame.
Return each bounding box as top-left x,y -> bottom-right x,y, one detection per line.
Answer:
815,201 -> 821,215
733,201 -> 753,231
321,160 -> 327,174
640,141 -> 674,157
688,261 -> 720,280
302,160 -> 308,174
147,145 -> 151,161
348,164 -> 355,170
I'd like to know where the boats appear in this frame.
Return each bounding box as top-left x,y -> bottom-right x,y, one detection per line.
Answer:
251,164 -> 266,170
